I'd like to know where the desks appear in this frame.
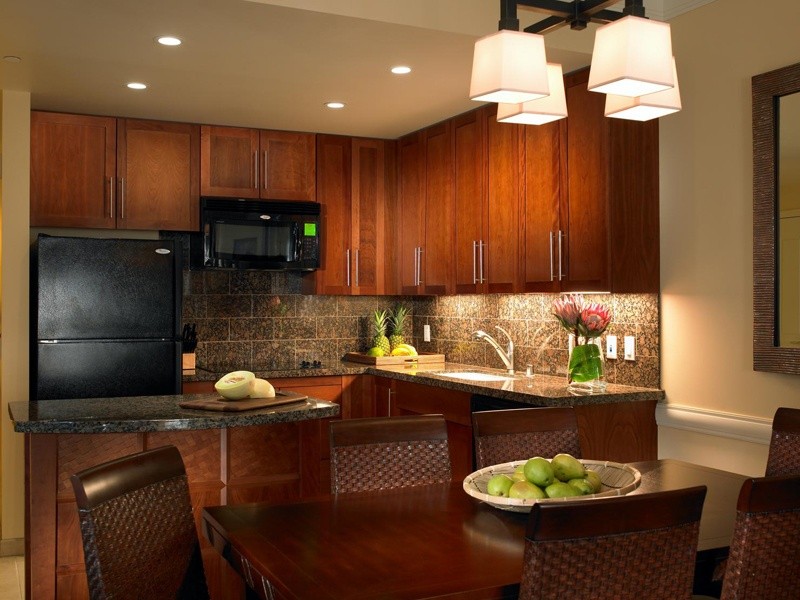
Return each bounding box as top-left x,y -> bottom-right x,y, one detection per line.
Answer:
202,459 -> 755,600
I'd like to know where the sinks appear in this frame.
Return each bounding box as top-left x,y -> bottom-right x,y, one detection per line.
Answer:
427,369 -> 521,383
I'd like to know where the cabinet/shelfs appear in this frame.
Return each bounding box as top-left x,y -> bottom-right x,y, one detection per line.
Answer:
29,109 -> 200,232
183,373 -> 473,482
200,124 -> 316,202
521,64 -> 660,295
456,101 -> 522,294
302,133 -> 395,296
396,116 -> 456,296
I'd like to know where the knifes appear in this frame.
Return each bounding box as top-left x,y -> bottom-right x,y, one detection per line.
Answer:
182,323 -> 198,352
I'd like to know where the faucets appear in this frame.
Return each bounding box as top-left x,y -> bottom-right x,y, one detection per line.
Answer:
473,326 -> 517,373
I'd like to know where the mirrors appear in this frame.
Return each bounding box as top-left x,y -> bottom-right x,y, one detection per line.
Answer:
750,62 -> 800,375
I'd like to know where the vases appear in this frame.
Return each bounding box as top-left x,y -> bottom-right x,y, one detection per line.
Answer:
424,324 -> 431,342
568,333 -> 606,396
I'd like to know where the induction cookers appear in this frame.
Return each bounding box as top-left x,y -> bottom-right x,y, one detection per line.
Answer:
198,360 -> 332,373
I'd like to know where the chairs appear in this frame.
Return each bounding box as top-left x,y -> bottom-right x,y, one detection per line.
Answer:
473,406 -> 581,470
765,407 -> 800,478
328,414 -> 451,494
719,473 -> 800,600
518,486 -> 708,600
70,445 -> 209,600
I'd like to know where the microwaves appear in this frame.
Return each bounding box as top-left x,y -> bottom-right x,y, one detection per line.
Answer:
191,196 -> 321,273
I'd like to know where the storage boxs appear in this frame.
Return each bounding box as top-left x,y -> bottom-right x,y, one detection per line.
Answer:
346,352 -> 445,365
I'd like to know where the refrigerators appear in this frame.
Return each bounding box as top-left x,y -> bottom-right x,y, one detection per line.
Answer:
38,234 -> 182,399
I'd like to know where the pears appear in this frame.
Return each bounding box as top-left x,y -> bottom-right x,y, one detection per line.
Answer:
368,347 -> 384,357
391,344 -> 418,356
488,453 -> 602,499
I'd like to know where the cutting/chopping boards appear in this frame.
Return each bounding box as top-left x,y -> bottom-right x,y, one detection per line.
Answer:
178,388 -> 310,411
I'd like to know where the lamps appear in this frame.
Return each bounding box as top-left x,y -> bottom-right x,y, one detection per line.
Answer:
470,0 -> 683,127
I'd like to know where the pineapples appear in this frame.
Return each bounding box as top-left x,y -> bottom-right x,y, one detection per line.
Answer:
371,308 -> 390,356
388,305 -> 411,351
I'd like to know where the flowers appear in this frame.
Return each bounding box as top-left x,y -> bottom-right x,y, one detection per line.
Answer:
549,294 -> 613,384
